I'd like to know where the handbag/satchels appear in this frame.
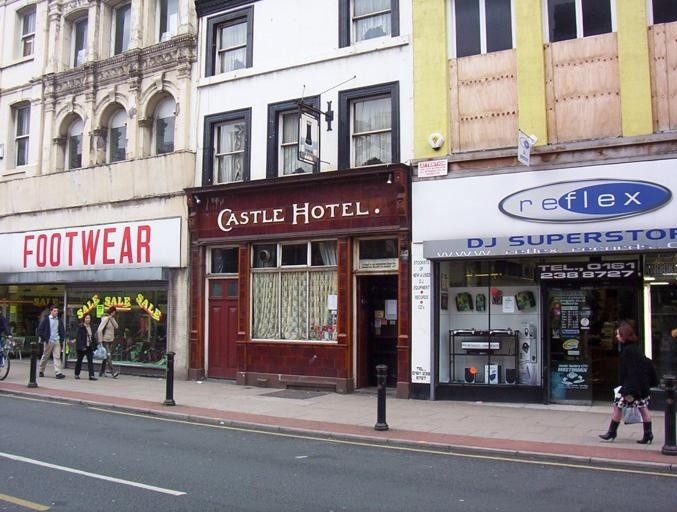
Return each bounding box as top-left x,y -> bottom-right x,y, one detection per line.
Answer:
622,407 -> 644,424
643,355 -> 659,387
93,345 -> 106,360
94,315 -> 110,344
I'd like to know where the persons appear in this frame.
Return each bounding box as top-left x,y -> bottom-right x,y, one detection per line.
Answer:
37,305 -> 66,379
598,319 -> 654,444
97,306 -> 120,378
74,313 -> 98,381
0,305 -> 15,367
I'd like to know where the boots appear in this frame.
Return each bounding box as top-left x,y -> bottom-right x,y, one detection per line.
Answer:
598,420 -> 620,442
636,421 -> 653,444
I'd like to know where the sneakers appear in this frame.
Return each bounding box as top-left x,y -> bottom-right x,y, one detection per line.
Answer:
56,373 -> 65,379
89,376 -> 97,380
39,372 -> 44,377
75,375 -> 78,379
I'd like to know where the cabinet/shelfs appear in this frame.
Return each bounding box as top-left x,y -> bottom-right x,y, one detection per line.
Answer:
448,329 -> 521,385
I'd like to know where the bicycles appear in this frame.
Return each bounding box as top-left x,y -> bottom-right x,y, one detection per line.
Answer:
0,335 -> 13,381
